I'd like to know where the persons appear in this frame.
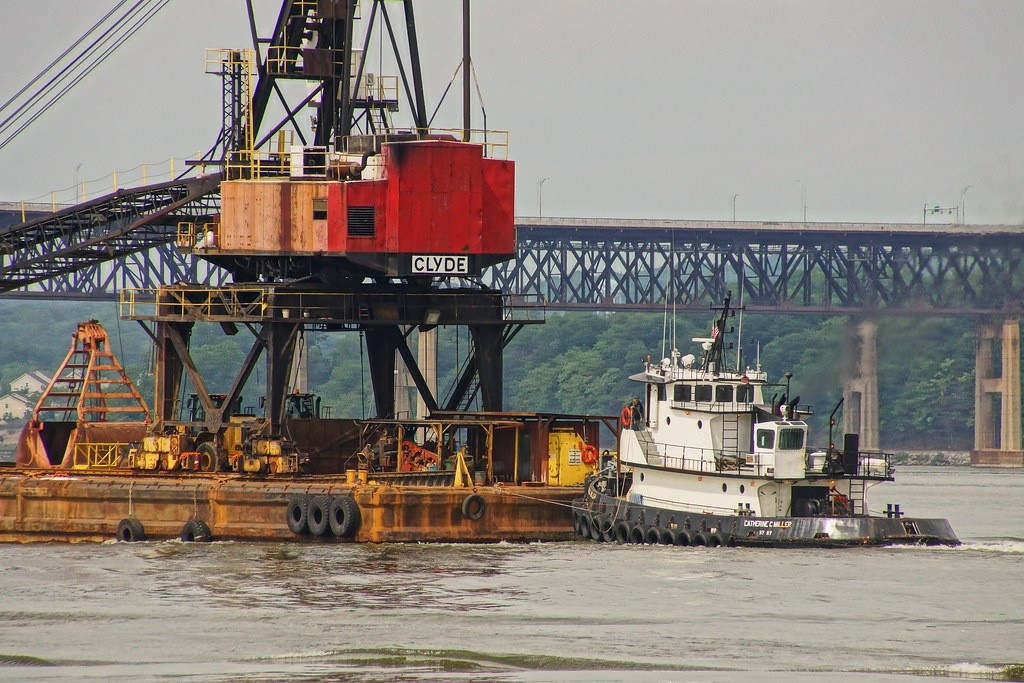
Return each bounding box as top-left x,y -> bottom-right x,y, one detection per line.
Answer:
628,397 -> 643,431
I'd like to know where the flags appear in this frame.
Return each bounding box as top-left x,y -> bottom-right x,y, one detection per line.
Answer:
711,317 -> 719,338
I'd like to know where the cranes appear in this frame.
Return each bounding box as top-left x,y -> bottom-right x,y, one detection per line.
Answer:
116,0 -> 546,469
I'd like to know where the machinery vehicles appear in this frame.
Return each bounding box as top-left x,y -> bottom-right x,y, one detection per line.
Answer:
257,389 -> 334,420
183,392 -> 256,475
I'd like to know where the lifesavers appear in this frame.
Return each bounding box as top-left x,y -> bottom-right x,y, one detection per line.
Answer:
571,509 -> 731,548
461,494 -> 486,520
580,444 -> 598,465
181,520 -> 212,542
116,517 -> 145,543
621,407 -> 631,427
286,494 -> 360,537
833,495 -> 847,510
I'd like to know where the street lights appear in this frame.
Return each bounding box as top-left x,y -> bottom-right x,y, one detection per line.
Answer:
922,202 -> 928,228
794,178 -> 807,223
539,177 -> 550,221
732,193 -> 740,224
74,163 -> 83,205
961,184 -> 976,224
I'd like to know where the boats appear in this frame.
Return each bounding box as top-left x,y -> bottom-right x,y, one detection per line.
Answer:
1,316 -> 626,543
571,287 -> 964,550
